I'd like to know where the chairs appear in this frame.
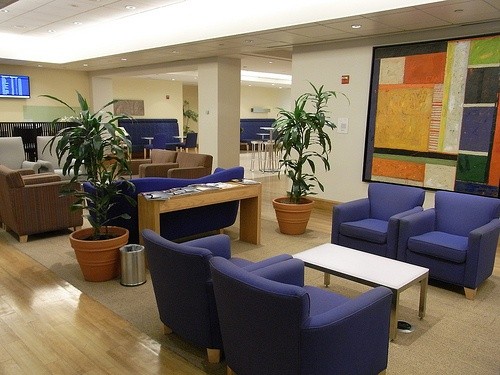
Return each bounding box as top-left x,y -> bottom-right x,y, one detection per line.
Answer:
144,132 -> 168,161
176,132 -> 198,153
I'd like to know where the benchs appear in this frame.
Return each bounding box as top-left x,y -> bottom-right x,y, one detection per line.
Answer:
240,118 -> 285,151
116,118 -> 181,160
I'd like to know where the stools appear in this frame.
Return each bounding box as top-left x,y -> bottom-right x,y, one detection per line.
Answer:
250,138 -> 290,175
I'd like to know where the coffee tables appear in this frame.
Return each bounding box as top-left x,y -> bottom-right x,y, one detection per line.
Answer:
291,242 -> 430,343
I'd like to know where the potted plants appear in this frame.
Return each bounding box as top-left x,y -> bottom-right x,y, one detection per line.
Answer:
35,89 -> 137,282
271,79 -> 351,235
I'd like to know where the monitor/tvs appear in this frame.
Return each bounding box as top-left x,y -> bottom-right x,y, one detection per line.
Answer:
0,73 -> 30,98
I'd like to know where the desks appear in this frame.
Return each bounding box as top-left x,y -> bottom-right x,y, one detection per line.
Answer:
137,178 -> 263,271
141,135 -> 159,146
256,132 -> 271,152
260,126 -> 281,173
173,135 -> 188,152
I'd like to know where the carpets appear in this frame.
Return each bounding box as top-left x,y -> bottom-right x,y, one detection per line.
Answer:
317,283 -> 442,348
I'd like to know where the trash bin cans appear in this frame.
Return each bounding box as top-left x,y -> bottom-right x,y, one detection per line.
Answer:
120,244 -> 146,287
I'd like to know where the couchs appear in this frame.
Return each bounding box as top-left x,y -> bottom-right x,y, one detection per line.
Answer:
0,168 -> 54,231
140,229 -> 293,365
0,164 -> 83,244
208,256 -> 393,375
0,136 -> 42,174
397,191 -> 500,300
331,182 -> 426,260
120,149 -> 179,180
83,166 -> 245,245
141,152 -> 213,179
37,136 -> 88,180
